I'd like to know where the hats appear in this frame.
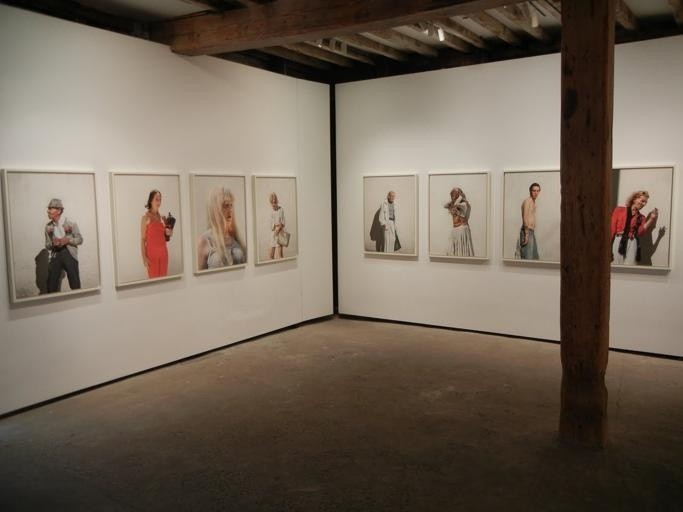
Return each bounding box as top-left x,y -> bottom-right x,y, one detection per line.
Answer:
47,199 -> 63,208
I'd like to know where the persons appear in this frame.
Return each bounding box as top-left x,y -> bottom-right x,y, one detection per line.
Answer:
268,191 -> 285,260
443,187 -> 475,256
44,199 -> 83,293
140,189 -> 174,277
520,183 -> 541,260
611,191 -> 657,266
197,185 -> 246,271
378,190 -> 400,253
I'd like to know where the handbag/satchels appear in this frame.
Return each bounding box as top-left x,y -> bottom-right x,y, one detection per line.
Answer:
278,232 -> 290,247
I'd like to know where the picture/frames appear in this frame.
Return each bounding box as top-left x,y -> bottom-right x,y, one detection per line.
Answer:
358,173 -> 419,260
502,170 -> 561,265
0,166 -> 102,311
427,170 -> 490,260
611,166 -> 672,271
189,172 -> 248,275
108,170 -> 186,290
252,174 -> 298,267
